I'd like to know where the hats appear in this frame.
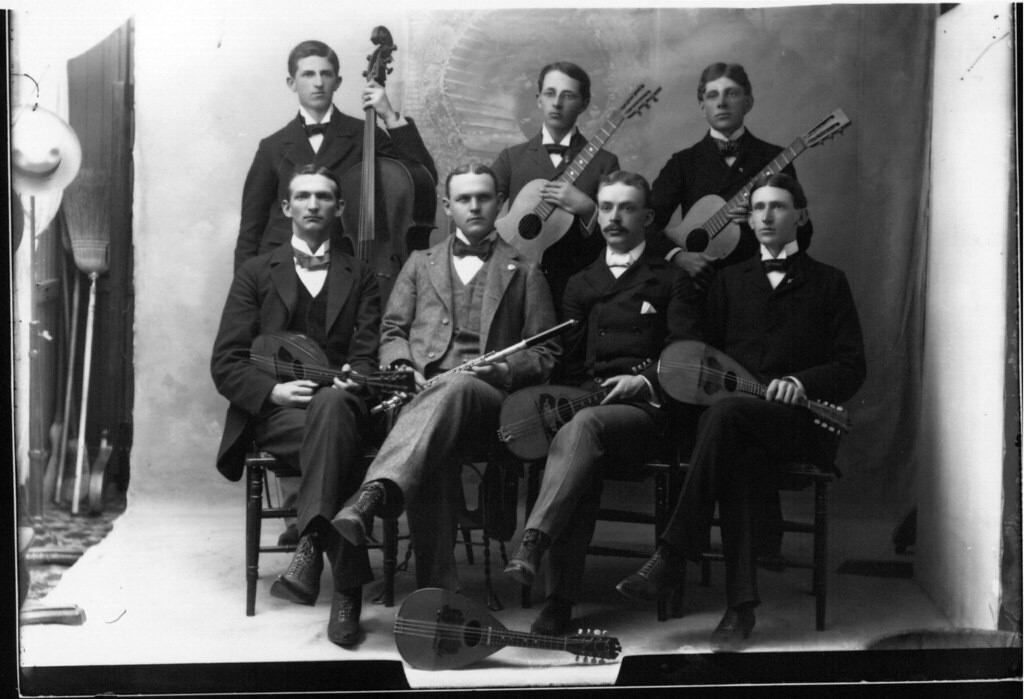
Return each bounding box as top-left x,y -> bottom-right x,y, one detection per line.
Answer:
12,106 -> 83,197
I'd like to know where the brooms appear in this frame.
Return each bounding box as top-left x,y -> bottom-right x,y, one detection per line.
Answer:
60,166 -> 112,515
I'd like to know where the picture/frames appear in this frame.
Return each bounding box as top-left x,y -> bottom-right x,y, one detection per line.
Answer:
1,1 -> 1024,699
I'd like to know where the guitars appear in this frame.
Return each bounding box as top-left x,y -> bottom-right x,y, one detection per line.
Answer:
393,587 -> 622,671
656,338 -> 851,437
497,356 -> 659,461
492,81 -> 663,264
246,330 -> 417,399
650,107 -> 855,268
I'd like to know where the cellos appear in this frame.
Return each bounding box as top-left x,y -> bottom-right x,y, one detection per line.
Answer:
339,22 -> 440,321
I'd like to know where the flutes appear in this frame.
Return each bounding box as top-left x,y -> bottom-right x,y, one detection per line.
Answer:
370,318 -> 578,416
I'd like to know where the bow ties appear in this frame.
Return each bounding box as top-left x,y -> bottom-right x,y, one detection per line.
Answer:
296,248 -> 331,269
607,254 -> 631,267
544,142 -> 568,158
299,114 -> 329,135
714,137 -> 741,156
763,259 -> 788,273
452,235 -> 492,262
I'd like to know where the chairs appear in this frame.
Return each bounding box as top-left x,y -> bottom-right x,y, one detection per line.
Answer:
242,439 -> 838,633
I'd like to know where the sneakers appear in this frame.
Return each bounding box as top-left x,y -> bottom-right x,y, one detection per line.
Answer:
269,543 -> 322,606
327,587 -> 363,645
530,598 -> 573,638
708,604 -> 756,652
616,545 -> 681,605
503,530 -> 548,586
331,485 -> 382,543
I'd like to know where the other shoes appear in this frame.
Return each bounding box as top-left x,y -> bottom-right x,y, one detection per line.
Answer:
279,525 -> 300,549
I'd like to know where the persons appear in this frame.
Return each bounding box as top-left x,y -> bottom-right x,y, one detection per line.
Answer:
646,60 -> 813,573
233,39 -> 439,287
210,162 -> 381,648
616,173 -> 868,654
486,62 -> 621,540
505,167 -> 706,638
330,159 -> 564,592
